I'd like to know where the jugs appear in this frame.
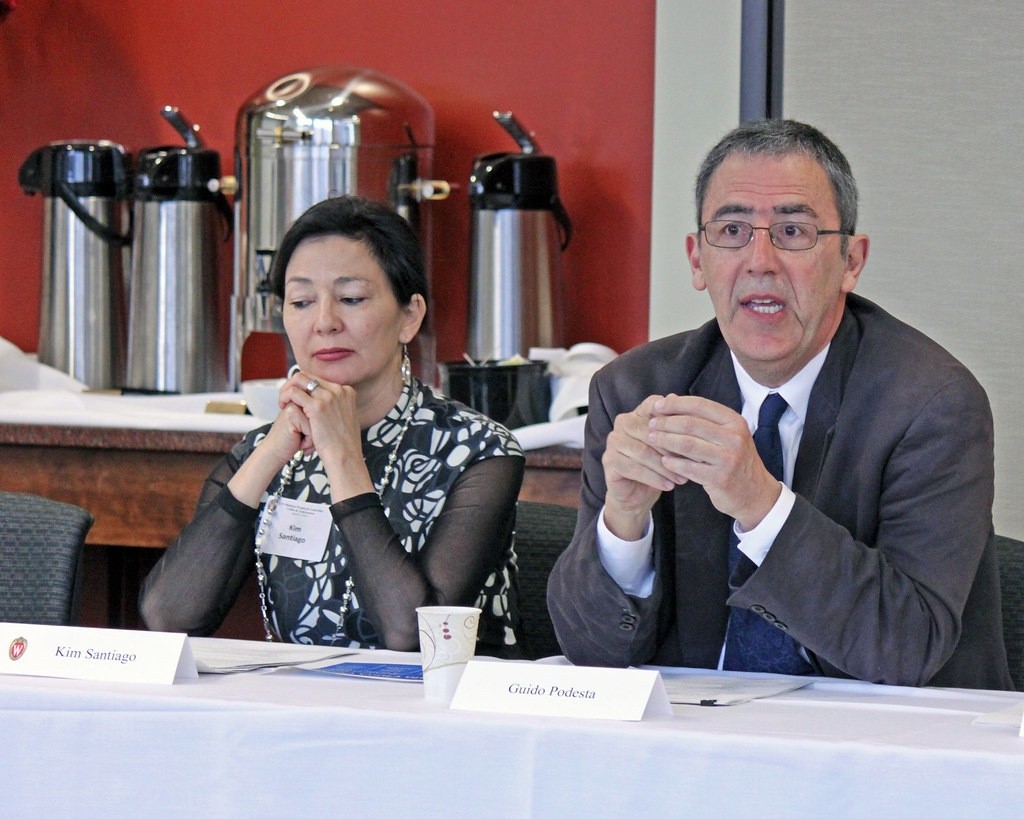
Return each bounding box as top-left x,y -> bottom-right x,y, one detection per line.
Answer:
19,106 -> 234,397
463,110 -> 575,362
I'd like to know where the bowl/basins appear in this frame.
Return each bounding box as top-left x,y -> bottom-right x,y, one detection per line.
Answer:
241,377 -> 288,419
437,357 -> 551,427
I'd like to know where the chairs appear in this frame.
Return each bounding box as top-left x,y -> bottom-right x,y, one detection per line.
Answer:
510,500 -> 580,655
0,490 -> 94,626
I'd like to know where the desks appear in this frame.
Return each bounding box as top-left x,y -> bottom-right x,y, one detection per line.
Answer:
0,621 -> 1024,819
0,422 -> 583,632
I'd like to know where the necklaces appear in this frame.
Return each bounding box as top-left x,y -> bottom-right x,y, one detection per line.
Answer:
255,376 -> 418,646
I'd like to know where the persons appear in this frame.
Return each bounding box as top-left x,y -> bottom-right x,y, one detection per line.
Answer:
137,194 -> 526,659
547,120 -> 1015,692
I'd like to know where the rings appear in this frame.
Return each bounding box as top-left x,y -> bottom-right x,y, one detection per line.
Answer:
306,379 -> 320,396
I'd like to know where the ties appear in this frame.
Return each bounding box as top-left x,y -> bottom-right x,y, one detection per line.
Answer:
721,391 -> 816,677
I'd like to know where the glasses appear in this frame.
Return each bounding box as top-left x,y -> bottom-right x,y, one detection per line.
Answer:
697,219 -> 852,252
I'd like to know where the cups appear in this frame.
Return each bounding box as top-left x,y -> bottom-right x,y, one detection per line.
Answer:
414,604 -> 483,708
